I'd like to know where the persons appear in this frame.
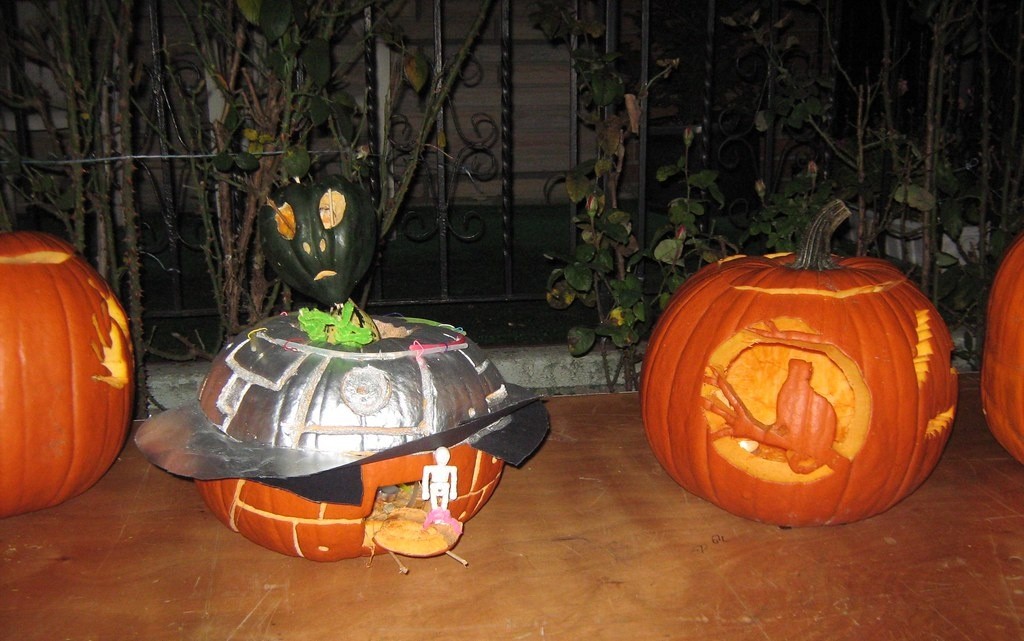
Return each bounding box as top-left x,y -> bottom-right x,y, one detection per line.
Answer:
421,446 -> 457,524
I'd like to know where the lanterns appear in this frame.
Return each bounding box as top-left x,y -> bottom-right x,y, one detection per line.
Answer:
639,251 -> 958,528
0,232 -> 135,520
258,174 -> 375,308
